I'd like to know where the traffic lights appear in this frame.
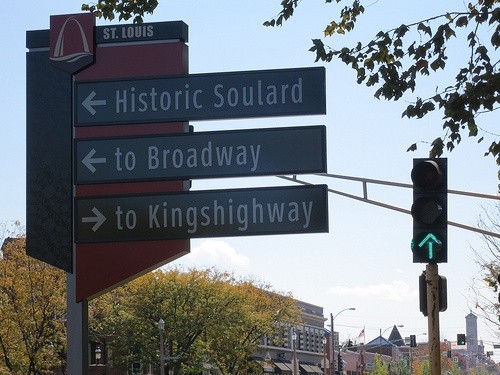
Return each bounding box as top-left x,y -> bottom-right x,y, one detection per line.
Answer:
410,156 -> 448,265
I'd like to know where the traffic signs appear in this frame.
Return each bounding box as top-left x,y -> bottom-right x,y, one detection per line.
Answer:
71,124 -> 329,186
71,64 -> 328,126
73,183 -> 331,245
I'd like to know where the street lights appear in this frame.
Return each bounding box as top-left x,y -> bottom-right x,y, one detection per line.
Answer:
330,307 -> 356,375
379,325 -> 405,365
